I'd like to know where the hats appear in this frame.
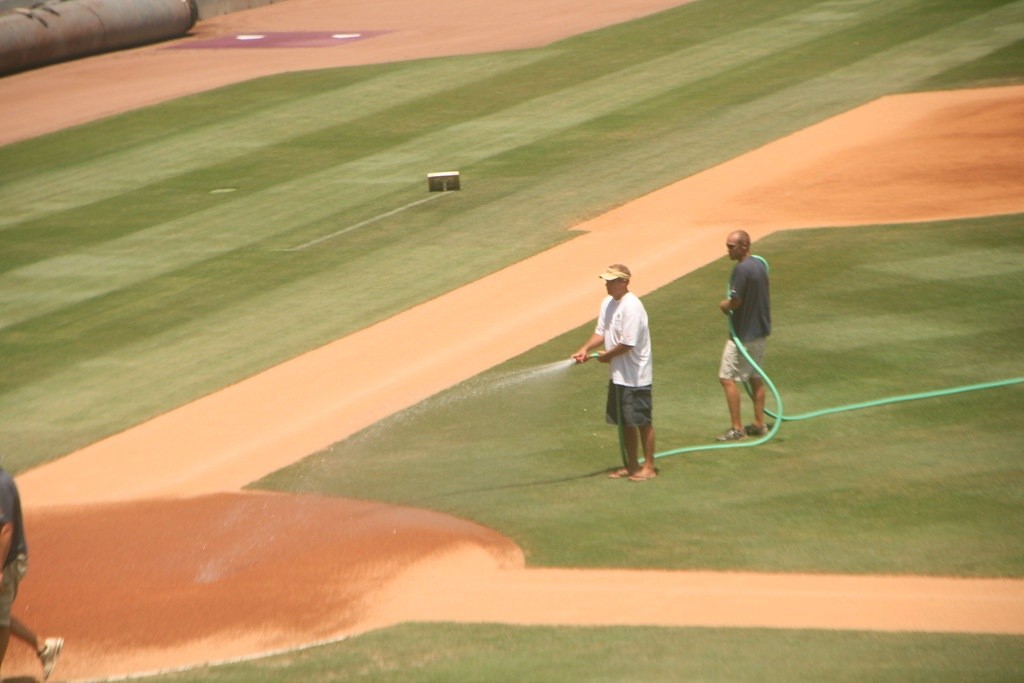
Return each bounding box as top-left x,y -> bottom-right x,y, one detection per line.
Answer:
599,267 -> 630,283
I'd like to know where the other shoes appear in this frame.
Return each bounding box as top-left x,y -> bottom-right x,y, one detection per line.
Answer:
630,467 -> 656,481
611,464 -> 640,478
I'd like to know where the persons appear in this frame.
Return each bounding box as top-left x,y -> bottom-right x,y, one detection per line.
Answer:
572,264 -> 656,481
717,230 -> 771,441
0,467 -> 64,683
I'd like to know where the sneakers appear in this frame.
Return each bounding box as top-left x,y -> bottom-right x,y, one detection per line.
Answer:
746,423 -> 768,436
717,427 -> 748,441
38,637 -> 63,681
